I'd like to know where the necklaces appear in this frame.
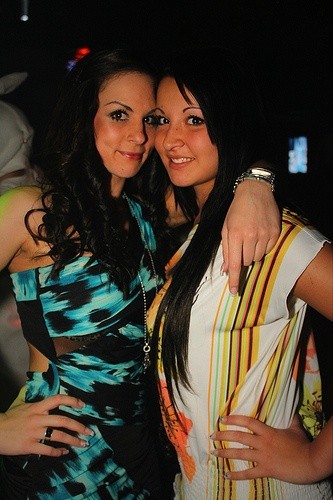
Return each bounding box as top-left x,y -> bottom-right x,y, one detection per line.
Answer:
113,192 -> 159,373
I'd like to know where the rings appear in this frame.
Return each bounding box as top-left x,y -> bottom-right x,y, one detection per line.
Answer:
45,426 -> 54,440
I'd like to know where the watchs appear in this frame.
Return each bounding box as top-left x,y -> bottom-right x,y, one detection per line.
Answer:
232,166 -> 276,195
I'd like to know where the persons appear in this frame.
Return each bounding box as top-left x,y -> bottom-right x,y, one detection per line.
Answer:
0,51 -> 186,499
143,57 -> 333,500
